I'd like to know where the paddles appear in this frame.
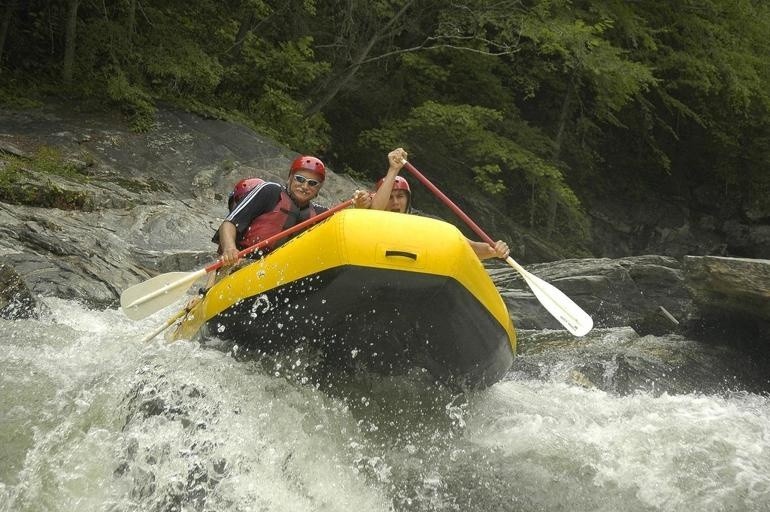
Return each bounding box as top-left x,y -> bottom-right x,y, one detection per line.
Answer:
119,189 -> 361,323
392,150 -> 594,337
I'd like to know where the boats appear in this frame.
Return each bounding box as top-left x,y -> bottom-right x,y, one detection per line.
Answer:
167,208 -> 520,406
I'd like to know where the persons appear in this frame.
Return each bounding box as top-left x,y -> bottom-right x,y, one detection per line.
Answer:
217,157 -> 371,269
230,178 -> 266,203
371,149 -> 509,263
207,190 -> 238,285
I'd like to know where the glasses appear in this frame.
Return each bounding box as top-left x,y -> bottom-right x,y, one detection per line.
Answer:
293,175 -> 320,187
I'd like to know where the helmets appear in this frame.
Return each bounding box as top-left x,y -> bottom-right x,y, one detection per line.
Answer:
376,175 -> 410,193
233,178 -> 265,202
289,156 -> 325,181
